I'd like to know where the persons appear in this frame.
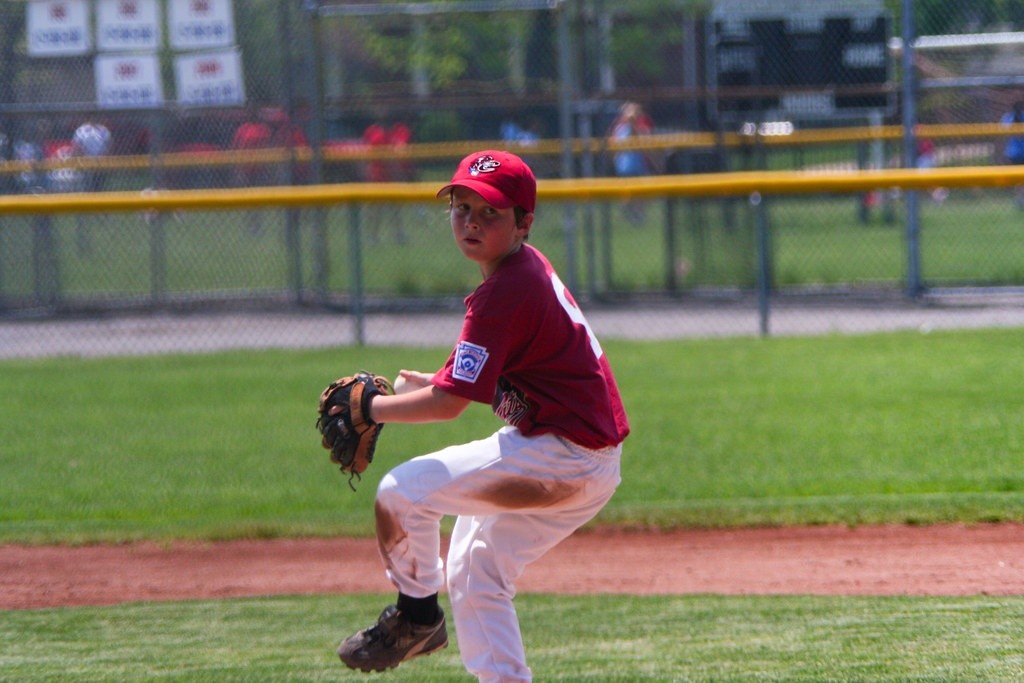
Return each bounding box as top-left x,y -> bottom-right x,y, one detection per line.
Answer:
503,104 -> 666,228
914,101 -> 1024,193
0,110 -> 416,243
315,151 -> 631,682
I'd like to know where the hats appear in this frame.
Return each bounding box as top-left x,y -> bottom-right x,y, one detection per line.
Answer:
436,151 -> 537,212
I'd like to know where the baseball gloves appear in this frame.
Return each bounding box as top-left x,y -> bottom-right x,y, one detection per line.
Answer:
315,370 -> 396,475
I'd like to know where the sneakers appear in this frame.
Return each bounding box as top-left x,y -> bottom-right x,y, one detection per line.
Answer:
338,604 -> 449,672
